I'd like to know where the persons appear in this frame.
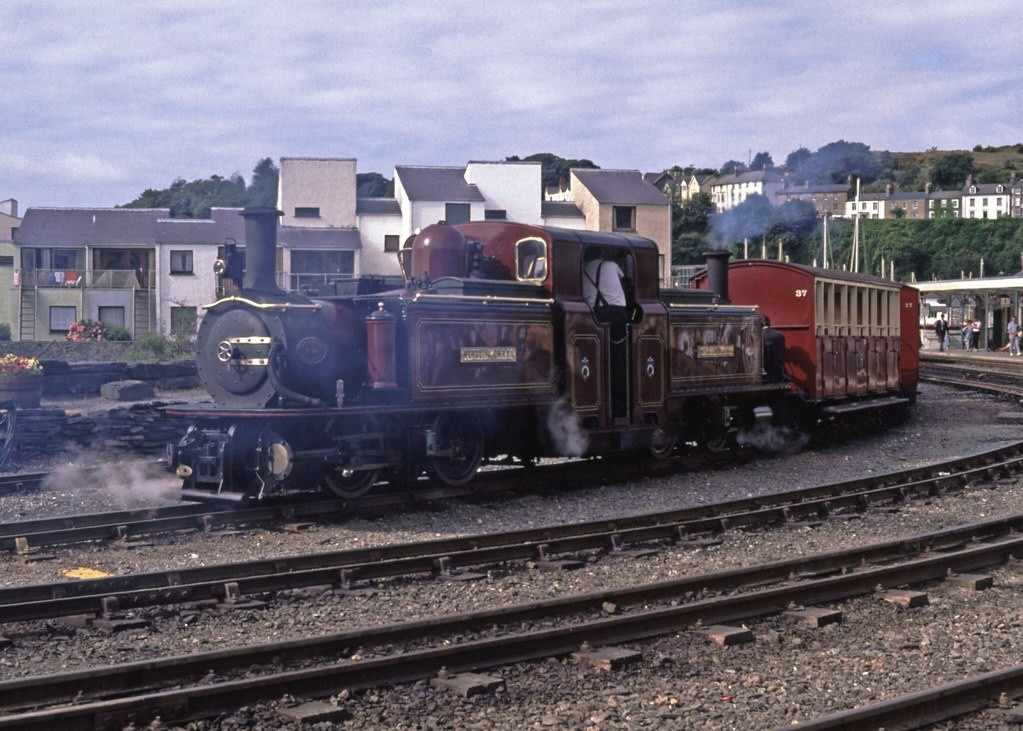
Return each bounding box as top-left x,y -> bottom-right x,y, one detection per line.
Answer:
1000,327 -> 1022,351
1007,315 -> 1022,357
962,319 -> 973,352
971,316 -> 982,352
935,314 -> 949,352
581,245 -> 630,417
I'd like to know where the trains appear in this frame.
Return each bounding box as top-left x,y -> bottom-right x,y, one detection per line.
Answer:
155,201 -> 923,517
